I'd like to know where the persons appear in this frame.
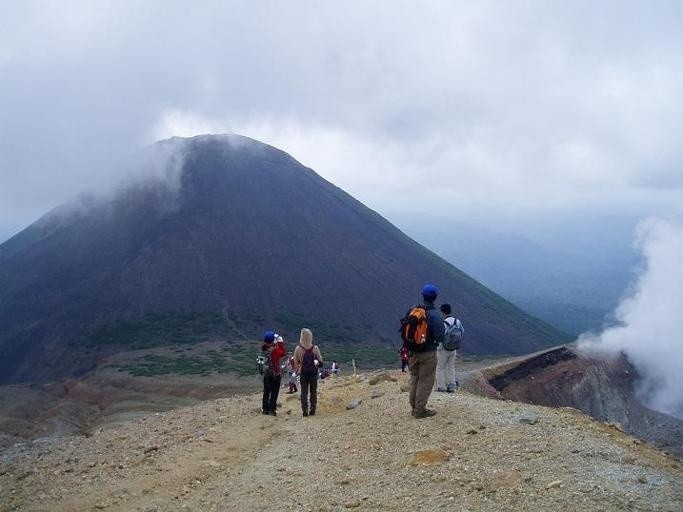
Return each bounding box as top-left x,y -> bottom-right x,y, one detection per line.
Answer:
398,344 -> 411,373
295,327 -> 322,416
408,283 -> 446,419
256,330 -> 287,416
281,351 -> 298,393
435,303 -> 465,394
321,360 -> 339,379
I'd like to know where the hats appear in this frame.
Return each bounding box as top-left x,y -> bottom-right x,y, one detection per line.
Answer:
264,332 -> 275,344
420,284 -> 438,294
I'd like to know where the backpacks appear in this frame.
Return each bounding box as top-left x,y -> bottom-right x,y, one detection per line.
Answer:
298,345 -> 318,375
442,319 -> 462,351
256,352 -> 274,375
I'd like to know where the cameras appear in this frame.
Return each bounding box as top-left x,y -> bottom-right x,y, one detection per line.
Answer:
274,334 -> 282,340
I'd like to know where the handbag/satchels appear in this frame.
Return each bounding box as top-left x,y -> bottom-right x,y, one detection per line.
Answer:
400,305 -> 435,346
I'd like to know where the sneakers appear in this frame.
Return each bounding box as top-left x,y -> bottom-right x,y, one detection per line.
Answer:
285,389 -> 297,394
262,409 -> 276,416
303,411 -> 314,416
415,410 -> 436,420
438,387 -> 456,393
